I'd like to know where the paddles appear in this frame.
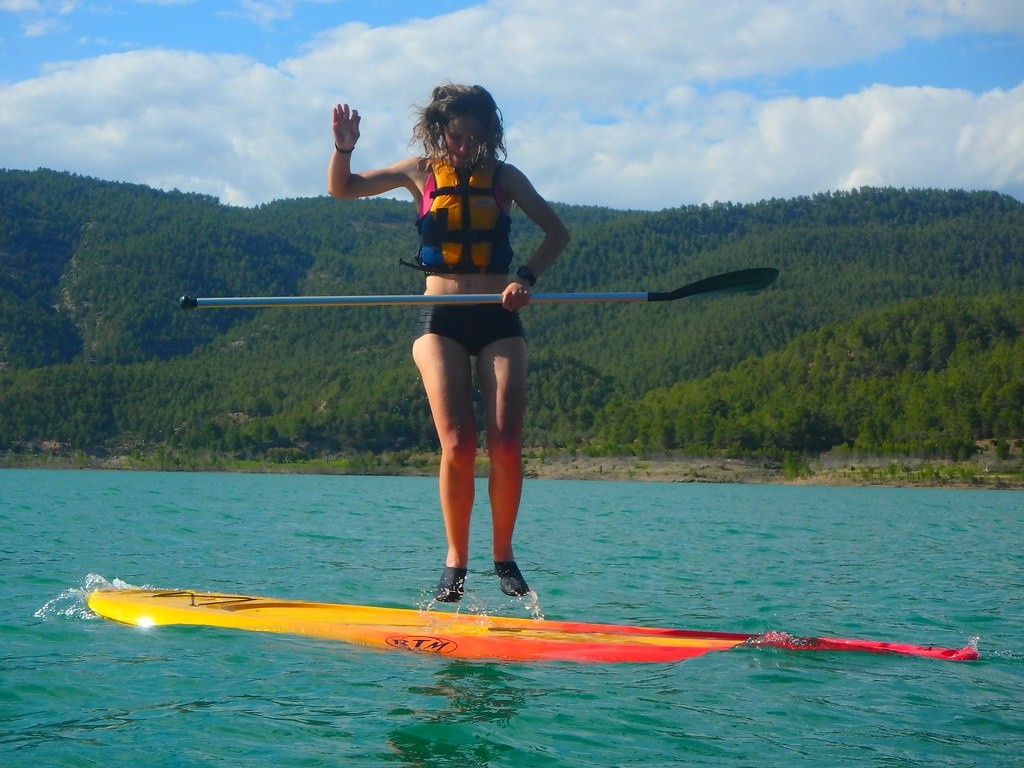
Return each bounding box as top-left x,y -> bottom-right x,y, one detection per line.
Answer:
177,267 -> 781,311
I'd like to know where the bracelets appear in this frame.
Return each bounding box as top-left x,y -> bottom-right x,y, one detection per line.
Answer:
335,142 -> 355,154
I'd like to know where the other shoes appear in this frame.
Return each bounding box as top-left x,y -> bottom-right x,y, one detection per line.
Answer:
493,558 -> 531,597
433,565 -> 467,604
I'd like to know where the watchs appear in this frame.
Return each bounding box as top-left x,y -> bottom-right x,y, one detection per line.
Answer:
516,266 -> 537,287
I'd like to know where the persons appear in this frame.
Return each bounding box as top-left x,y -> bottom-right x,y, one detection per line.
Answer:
328,84 -> 572,602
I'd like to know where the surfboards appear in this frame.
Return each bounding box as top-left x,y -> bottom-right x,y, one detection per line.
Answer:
88,586 -> 977,664
